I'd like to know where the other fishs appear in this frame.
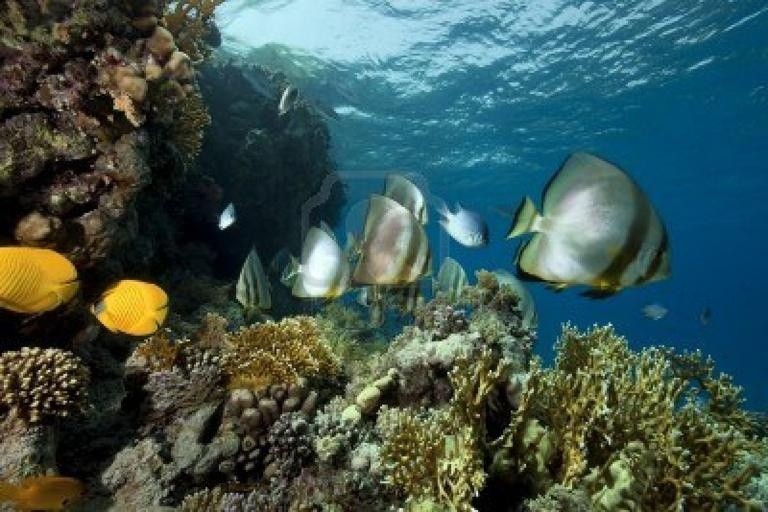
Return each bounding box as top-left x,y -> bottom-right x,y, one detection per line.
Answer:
490,267 -> 539,329
277,85 -> 300,116
0,473 -> 85,512
217,174 -> 486,329
641,304 -> 670,324
504,150 -> 673,300
88,279 -> 170,337
0,245 -> 81,317
